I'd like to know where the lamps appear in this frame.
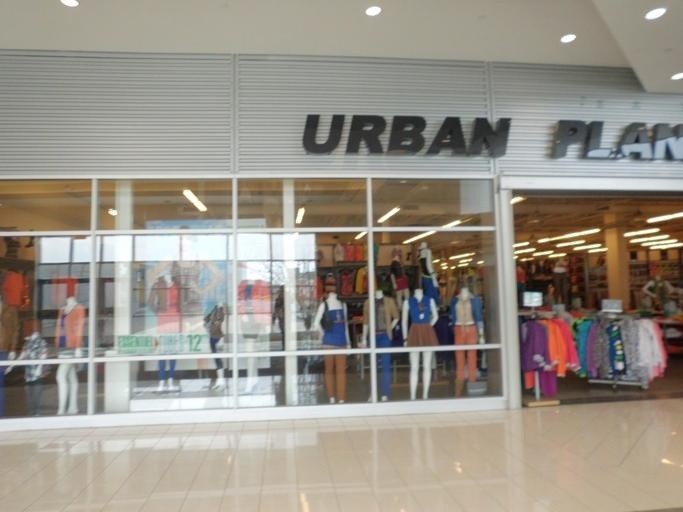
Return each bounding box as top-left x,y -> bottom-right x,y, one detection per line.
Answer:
523,291 -> 543,313
600,298 -> 623,317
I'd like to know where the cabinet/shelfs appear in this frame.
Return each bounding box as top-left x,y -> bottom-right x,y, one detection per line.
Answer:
314,260 -> 422,341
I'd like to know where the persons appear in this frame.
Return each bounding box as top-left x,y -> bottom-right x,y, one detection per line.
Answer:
542,283 -> 563,305
416,242 -> 440,307
401,288 -> 439,400
314,292 -> 352,404
391,255 -> 410,310
0,295 -> 48,416
203,303 -> 226,392
361,290 -> 400,402
272,285 -> 285,347
642,275 -> 674,305
54,296 -> 86,415
146,226 -> 225,333
147,271 -> 181,390
237,278 -> 271,395
448,287 -> 486,397
